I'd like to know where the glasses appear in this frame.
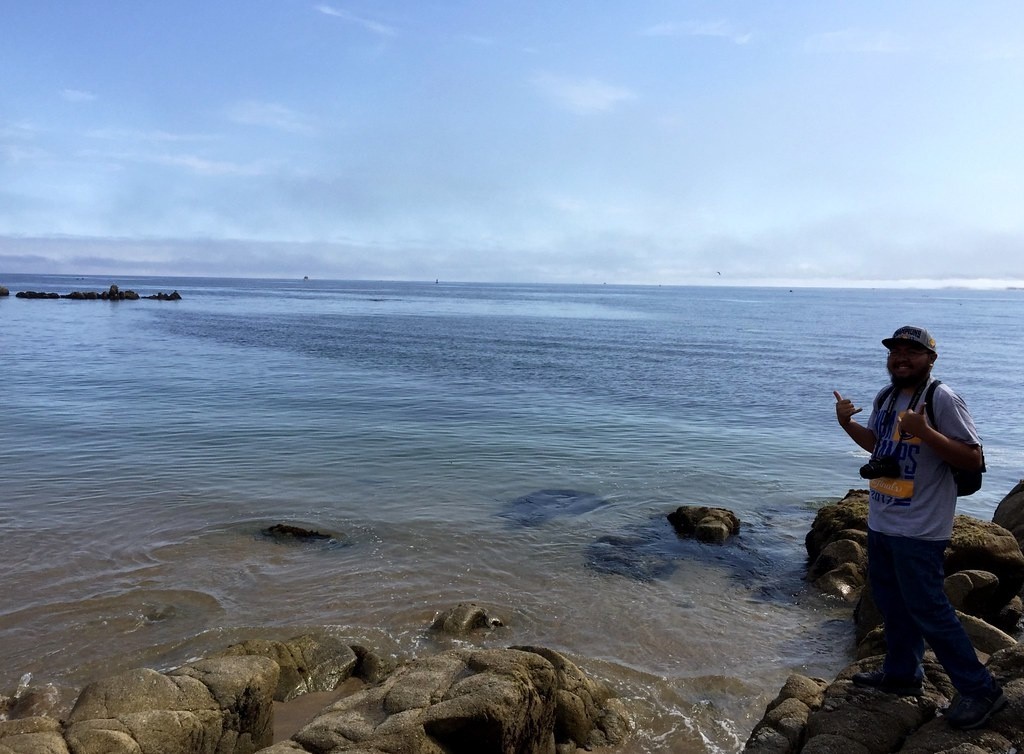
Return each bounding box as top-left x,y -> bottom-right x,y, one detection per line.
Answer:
887,349 -> 930,359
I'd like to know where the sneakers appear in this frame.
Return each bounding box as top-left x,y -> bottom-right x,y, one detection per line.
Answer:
946,677 -> 1005,729
851,669 -> 924,696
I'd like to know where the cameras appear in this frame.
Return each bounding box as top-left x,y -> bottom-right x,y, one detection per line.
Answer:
860,456 -> 884,480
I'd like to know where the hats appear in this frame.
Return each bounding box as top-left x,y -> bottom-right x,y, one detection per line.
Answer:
882,326 -> 938,364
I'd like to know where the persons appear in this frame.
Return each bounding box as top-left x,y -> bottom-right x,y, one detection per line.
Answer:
833,325 -> 1007,727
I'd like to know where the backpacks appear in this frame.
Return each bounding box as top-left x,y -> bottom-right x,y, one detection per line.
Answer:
878,380 -> 987,496
